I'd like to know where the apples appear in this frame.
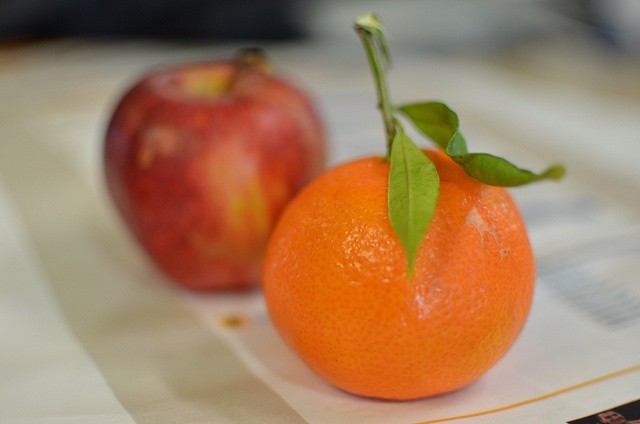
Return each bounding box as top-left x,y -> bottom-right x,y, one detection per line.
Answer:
100,46 -> 328,294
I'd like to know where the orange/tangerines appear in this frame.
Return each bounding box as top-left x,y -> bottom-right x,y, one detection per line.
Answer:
258,145 -> 536,401
